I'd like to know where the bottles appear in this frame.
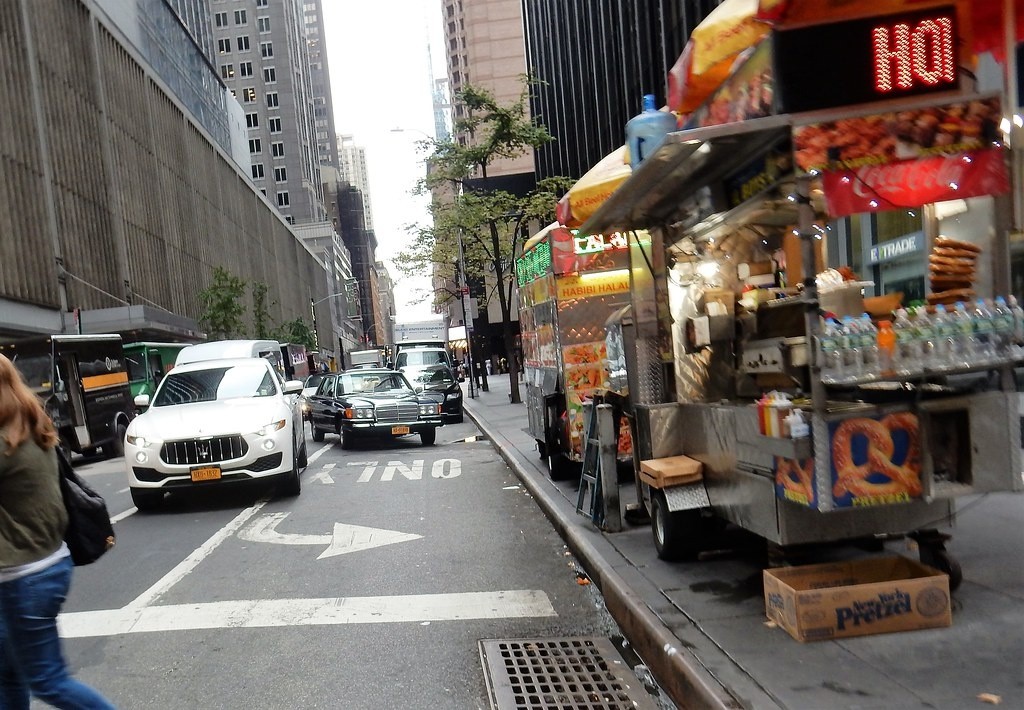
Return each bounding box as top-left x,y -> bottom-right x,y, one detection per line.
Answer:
822,317 -> 840,368
911,307 -> 936,356
839,315 -> 859,366
994,296 -> 1014,353
758,391 -> 793,438
626,94 -> 677,172
858,313 -> 878,365
1007,294 -> 1024,347
932,304 -> 951,356
972,298 -> 994,354
876,320 -> 897,377
891,308 -> 913,358
951,301 -> 973,354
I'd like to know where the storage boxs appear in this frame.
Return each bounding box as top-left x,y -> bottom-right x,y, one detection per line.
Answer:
764,552 -> 953,644
639,473 -> 702,489
639,455 -> 702,478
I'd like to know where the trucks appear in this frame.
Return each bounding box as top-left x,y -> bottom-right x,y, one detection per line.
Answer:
345,349 -> 388,371
0,333 -> 139,459
278,342 -> 311,396
121,341 -> 194,418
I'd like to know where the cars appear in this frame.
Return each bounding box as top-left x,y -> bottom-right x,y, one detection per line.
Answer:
396,362 -> 465,423
298,372 -> 333,420
125,357 -> 309,513
306,367 -> 446,450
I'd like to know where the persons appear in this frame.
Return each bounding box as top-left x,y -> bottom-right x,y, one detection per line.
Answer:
316,361 -> 329,373
0,351 -> 117,710
457,354 -> 508,382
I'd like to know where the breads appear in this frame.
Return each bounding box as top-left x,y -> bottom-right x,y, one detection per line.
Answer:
831,412 -> 922,498
773,456 -> 814,504
925,236 -> 981,313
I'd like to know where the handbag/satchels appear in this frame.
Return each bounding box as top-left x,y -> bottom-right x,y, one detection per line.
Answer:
54,445 -> 115,566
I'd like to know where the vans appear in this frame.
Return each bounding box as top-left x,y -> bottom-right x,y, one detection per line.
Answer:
175,339 -> 286,396
386,347 -> 461,373
